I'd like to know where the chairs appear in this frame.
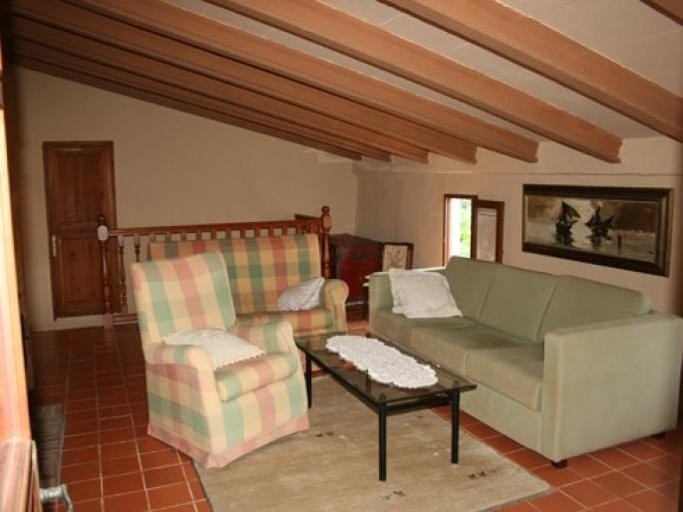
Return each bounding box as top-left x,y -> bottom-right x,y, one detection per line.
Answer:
130,250 -> 310,468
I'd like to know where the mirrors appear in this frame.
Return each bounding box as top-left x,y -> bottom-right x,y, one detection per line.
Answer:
472,200 -> 504,263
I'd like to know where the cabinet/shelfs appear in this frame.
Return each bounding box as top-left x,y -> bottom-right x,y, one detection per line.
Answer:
328,233 -> 383,306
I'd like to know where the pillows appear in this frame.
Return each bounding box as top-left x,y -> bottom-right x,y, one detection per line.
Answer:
277,276 -> 325,311
162,328 -> 265,371
396,272 -> 463,319
389,267 -> 421,315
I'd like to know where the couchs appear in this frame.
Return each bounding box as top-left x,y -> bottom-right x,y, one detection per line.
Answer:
367,257 -> 683,469
148,233 -> 349,375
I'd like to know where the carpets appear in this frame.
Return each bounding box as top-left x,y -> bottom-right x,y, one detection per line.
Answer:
190,375 -> 553,512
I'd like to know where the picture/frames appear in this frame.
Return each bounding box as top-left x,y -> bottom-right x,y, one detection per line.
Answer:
521,184 -> 674,277
383,242 -> 413,271
